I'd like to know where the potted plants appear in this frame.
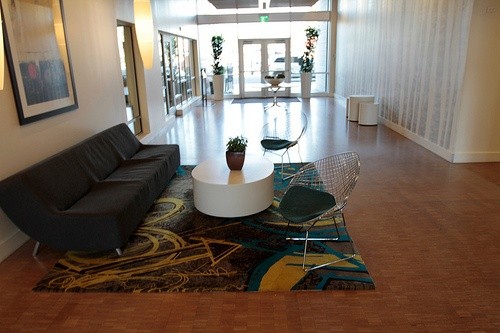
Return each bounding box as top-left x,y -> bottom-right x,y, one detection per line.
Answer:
211,35 -> 226,101
298,26 -> 320,99
226,136 -> 248,171
264,74 -> 285,85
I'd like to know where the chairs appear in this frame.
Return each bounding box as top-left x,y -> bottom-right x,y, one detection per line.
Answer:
260,108 -> 307,181
278,152 -> 361,271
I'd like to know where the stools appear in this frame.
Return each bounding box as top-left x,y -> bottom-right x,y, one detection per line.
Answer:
359,103 -> 379,125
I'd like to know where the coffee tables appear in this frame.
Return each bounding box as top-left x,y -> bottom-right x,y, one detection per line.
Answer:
191,158 -> 275,218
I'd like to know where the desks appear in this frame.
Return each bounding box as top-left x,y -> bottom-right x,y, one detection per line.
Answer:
349,96 -> 374,122
268,86 -> 283,107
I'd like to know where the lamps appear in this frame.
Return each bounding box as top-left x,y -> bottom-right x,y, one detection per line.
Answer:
133,0 -> 154,70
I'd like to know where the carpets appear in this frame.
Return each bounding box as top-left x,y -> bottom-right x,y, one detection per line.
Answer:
231,97 -> 301,103
33,162 -> 375,292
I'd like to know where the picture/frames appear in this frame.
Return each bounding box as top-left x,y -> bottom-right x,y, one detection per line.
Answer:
0,0 -> 79,126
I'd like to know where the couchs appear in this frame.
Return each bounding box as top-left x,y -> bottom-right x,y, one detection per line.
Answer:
0,123 -> 180,257
